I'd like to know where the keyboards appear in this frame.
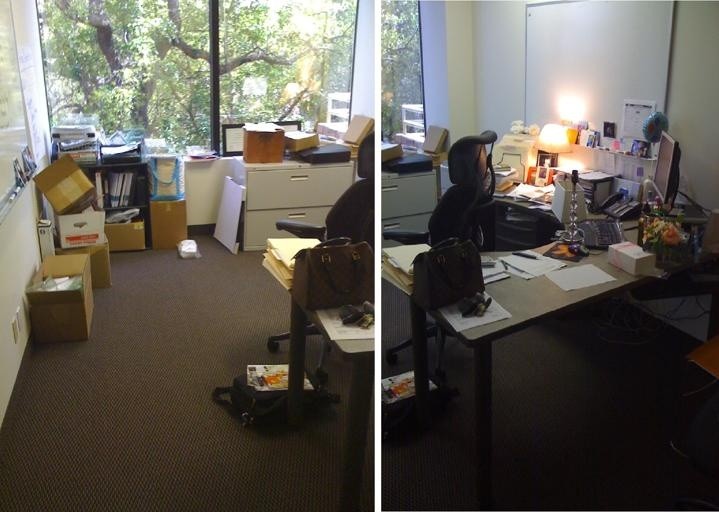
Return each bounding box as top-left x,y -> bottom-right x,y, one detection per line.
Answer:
584,219 -> 626,248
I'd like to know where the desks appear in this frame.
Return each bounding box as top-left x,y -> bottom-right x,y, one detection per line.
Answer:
410,230 -> 719,510
288,289 -> 376,428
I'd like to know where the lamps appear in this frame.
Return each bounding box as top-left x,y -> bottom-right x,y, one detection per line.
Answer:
534,124 -> 572,185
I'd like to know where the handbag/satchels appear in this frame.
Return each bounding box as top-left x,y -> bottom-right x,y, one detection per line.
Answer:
381,364 -> 460,435
290,235 -> 374,313
408,236 -> 486,312
211,366 -> 332,433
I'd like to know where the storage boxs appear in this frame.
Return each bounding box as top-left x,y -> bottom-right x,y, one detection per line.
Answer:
54,204 -> 106,249
243,125 -> 285,164
33,154 -> 97,215
676,336 -> 719,440
55,231 -> 113,289
149,200 -> 188,251
25,254 -> 95,346
104,218 -> 146,252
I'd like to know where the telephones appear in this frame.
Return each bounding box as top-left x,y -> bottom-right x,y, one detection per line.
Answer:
598,187 -> 642,218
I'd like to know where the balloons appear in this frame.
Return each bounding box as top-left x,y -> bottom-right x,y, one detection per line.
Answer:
643,112 -> 669,143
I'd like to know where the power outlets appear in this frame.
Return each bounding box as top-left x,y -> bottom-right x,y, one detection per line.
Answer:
12,317 -> 18,344
17,306 -> 23,332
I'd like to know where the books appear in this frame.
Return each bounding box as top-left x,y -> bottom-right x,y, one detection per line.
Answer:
488,164 -> 520,191
381,243 -> 432,294
422,125 -> 448,153
63,153 -> 97,165
342,115 -> 374,145
95,169 -> 133,209
381,369 -> 438,405
247,364 -> 314,392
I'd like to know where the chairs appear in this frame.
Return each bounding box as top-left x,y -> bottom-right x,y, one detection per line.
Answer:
267,131 -> 375,353
382,132 -> 498,366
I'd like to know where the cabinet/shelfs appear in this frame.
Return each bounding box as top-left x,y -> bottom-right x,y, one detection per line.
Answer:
382,171 -> 438,235
496,185 -> 640,237
234,156 -> 354,251
327,92 -> 350,123
52,155 -> 149,251
402,104 -> 426,134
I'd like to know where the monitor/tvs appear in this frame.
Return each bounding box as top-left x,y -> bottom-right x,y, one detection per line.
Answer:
651,129 -> 682,203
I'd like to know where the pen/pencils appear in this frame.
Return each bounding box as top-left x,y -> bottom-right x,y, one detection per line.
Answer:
499,257 -> 508,273
511,251 -> 541,260
